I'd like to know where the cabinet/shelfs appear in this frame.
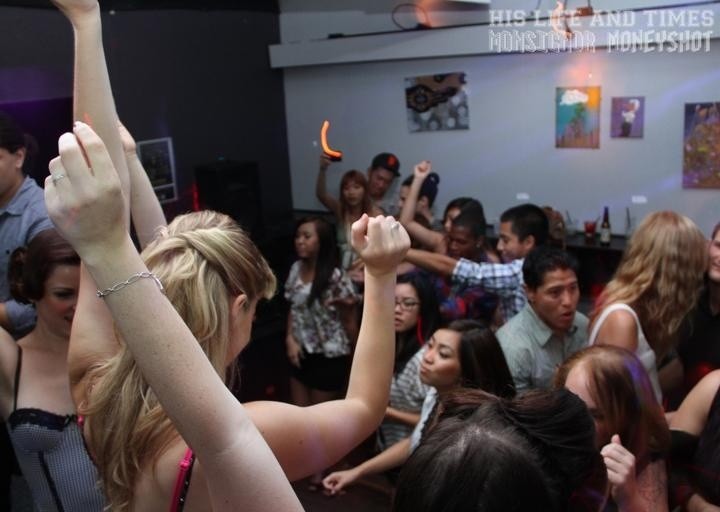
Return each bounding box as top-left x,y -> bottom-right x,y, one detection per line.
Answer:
570,231 -> 631,302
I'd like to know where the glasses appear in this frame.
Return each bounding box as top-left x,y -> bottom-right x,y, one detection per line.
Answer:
394,298 -> 420,312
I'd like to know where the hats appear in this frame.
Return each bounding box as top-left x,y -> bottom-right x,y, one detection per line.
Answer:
372,153 -> 400,176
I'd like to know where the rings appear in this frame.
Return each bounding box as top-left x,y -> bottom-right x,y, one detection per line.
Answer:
388,223 -> 401,230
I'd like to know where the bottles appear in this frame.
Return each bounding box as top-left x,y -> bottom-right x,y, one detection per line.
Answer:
600,206 -> 611,246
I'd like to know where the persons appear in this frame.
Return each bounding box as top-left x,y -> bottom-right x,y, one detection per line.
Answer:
49,0 -> 411,511
0,118 -> 720,511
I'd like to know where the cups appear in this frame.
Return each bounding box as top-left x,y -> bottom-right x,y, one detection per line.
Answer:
564,219 -> 580,236
584,221 -> 597,238
625,215 -> 636,242
